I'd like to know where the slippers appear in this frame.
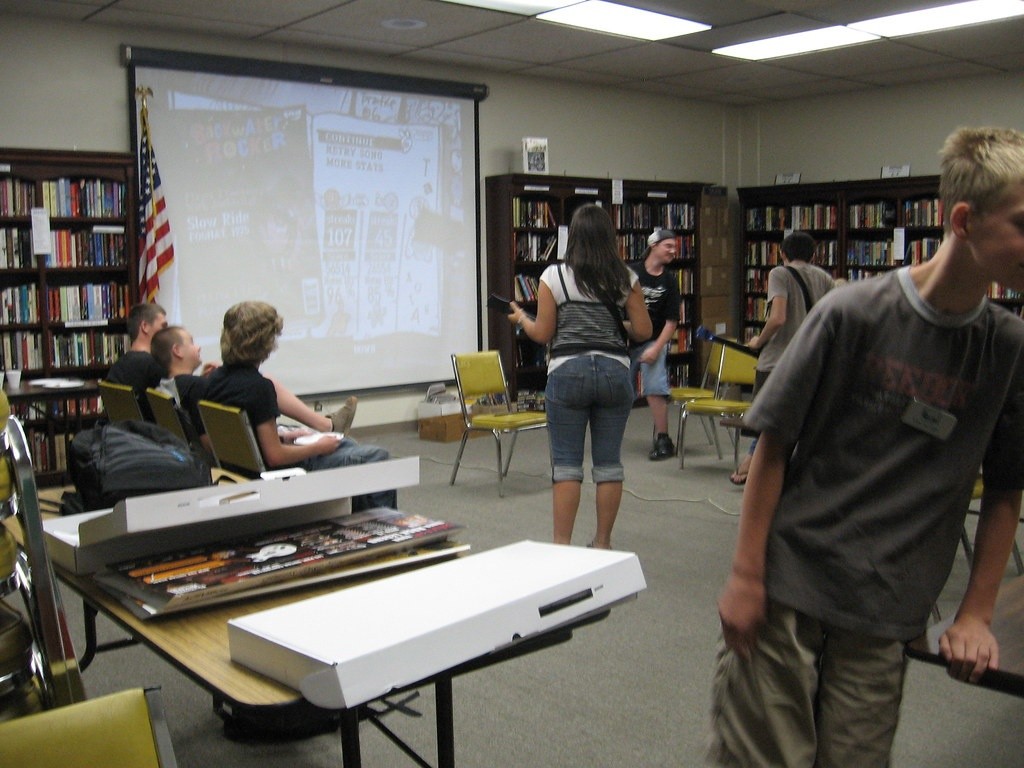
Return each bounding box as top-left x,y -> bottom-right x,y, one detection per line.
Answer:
729,468 -> 749,485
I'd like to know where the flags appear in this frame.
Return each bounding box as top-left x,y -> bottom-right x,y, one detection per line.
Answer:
137,87 -> 175,304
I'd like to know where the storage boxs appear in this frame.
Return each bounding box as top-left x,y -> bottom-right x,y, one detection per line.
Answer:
39,455 -> 420,574
227,539 -> 646,709
418,402 -> 493,444
699,186 -> 739,373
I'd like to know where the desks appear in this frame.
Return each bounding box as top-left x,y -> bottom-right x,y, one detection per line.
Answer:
1,469 -> 610,768
905,574 -> 1023,699
4,380 -> 101,477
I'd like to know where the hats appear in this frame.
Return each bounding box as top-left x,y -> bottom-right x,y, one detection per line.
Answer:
647,228 -> 675,248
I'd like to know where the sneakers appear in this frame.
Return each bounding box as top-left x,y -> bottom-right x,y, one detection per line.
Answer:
326,395 -> 357,435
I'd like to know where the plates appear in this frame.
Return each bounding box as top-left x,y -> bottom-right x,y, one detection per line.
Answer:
294,431 -> 345,445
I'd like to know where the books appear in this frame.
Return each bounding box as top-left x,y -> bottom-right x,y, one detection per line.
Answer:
743,198 -> 1024,344
93,511 -> 474,622
0,176 -> 125,218
0,283 -> 130,327
9,395 -> 104,476
0,225 -> 126,268
0,332 -> 131,370
513,196 -> 696,388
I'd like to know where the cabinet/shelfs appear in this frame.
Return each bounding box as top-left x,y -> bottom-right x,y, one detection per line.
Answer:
736,175 -> 1024,394
0,146 -> 139,489
486,173 -> 706,413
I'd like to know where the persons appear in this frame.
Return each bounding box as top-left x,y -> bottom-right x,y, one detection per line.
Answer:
149,301 -> 399,509
507,204 -> 653,550
105,304 -> 168,423
627,231 -> 682,462
706,126 -> 1024,768
730,233 -> 835,484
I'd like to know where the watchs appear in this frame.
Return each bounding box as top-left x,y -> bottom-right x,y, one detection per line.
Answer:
516,315 -> 526,330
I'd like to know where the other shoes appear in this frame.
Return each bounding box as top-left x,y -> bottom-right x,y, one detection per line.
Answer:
648,433 -> 674,461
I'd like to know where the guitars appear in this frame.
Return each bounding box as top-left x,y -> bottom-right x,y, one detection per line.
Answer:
695,325 -> 761,358
485,292 -> 536,322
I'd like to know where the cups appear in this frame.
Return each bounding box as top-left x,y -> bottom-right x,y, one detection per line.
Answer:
6,370 -> 21,389
0,373 -> 4,389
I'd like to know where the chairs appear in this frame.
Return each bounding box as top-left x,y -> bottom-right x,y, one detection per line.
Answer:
0,389 -> 180,768
449,349 -> 547,497
97,377 -> 143,424
961,471 -> 1024,577
0,685 -> 179,768
145,387 -> 190,452
669,337 -> 772,471
198,398 -> 266,481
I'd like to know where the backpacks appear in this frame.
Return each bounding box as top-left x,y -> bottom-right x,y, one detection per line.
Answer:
57,420 -> 213,514
